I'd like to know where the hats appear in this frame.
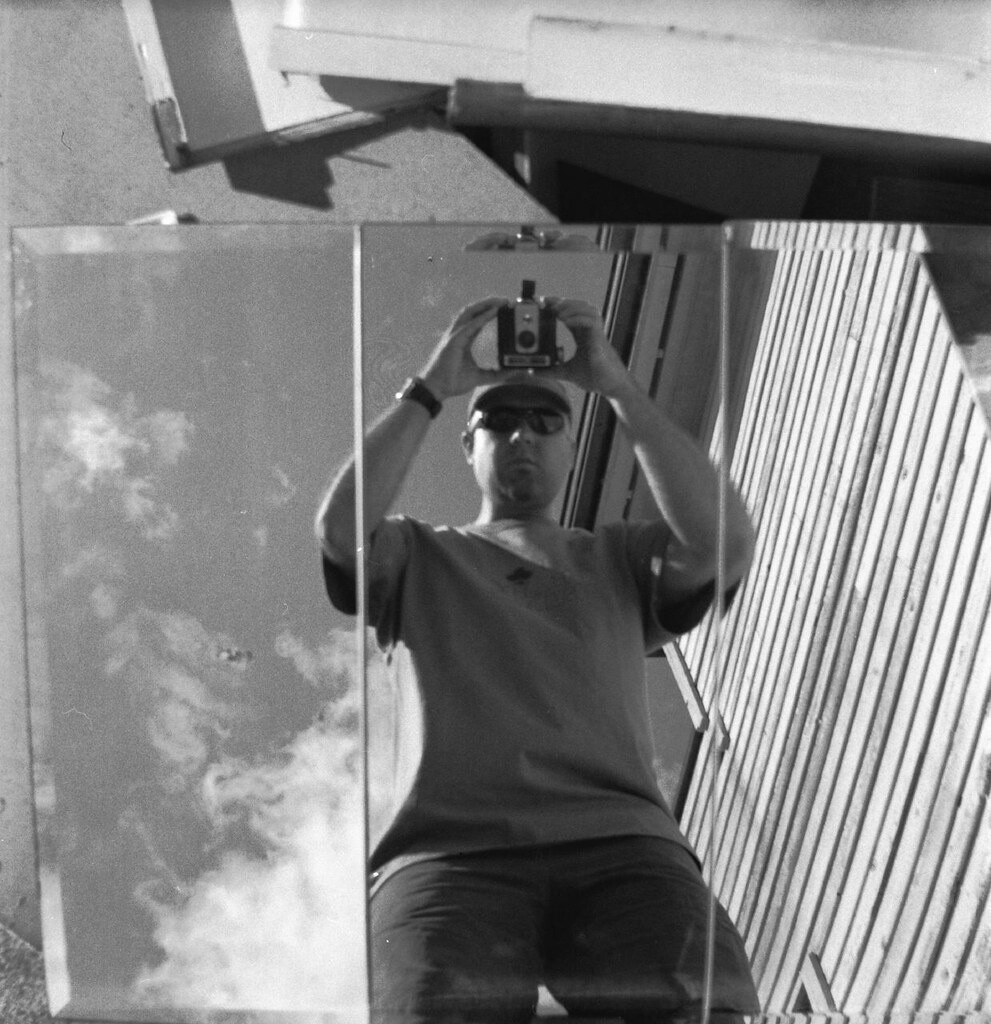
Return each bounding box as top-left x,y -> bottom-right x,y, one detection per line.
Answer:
469,377 -> 573,424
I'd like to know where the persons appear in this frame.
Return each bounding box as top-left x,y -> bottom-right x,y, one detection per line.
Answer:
315,295 -> 763,1024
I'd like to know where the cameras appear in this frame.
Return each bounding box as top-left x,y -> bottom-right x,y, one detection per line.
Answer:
495,280 -> 558,369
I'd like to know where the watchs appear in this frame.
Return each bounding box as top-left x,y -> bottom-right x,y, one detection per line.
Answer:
396,377 -> 442,418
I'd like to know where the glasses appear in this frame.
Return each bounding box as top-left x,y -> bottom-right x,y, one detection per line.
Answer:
467,403 -> 570,435
